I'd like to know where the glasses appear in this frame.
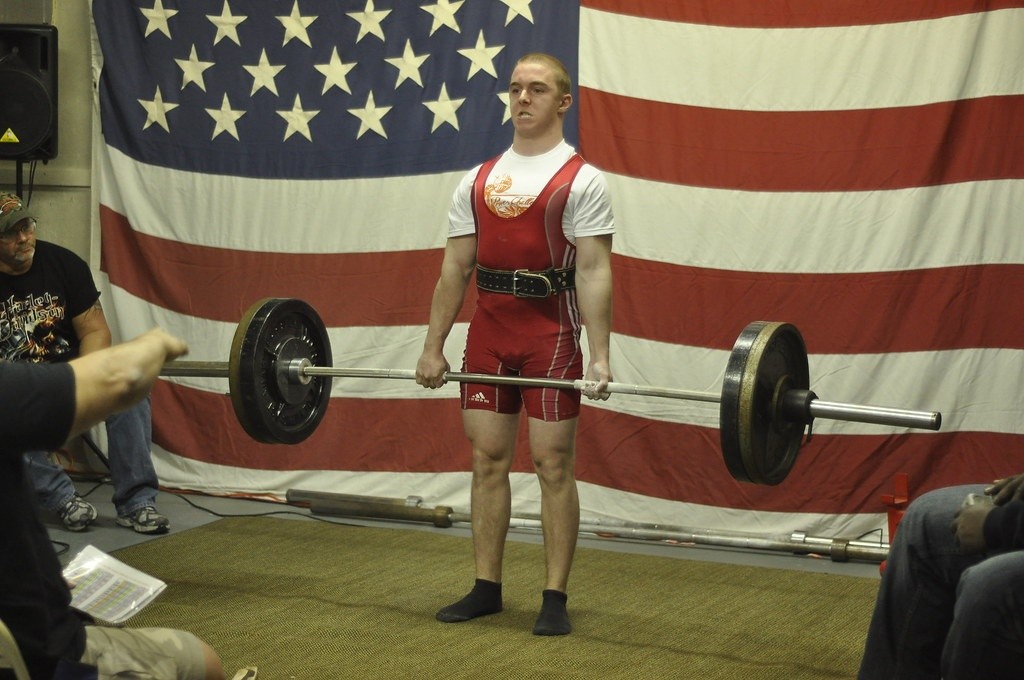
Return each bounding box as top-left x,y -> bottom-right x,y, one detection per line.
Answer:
0,218 -> 37,244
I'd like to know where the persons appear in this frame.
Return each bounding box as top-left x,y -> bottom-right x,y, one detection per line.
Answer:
416,54 -> 618,637
857,475 -> 1024,679
0,192 -> 222,679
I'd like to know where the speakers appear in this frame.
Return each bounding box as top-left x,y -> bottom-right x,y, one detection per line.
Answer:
0,23 -> 59,161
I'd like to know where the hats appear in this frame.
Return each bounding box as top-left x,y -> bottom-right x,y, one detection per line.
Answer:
0,191 -> 33,232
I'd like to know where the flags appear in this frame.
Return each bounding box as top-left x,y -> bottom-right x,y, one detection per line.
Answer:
85,0 -> 1024,548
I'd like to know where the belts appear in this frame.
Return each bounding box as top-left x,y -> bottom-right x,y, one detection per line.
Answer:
475,264 -> 575,299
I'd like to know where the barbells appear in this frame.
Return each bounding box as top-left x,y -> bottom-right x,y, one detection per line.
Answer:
157,297 -> 944,486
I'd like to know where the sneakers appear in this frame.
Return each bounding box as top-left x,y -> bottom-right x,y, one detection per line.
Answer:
116,506 -> 170,533
57,493 -> 97,531
